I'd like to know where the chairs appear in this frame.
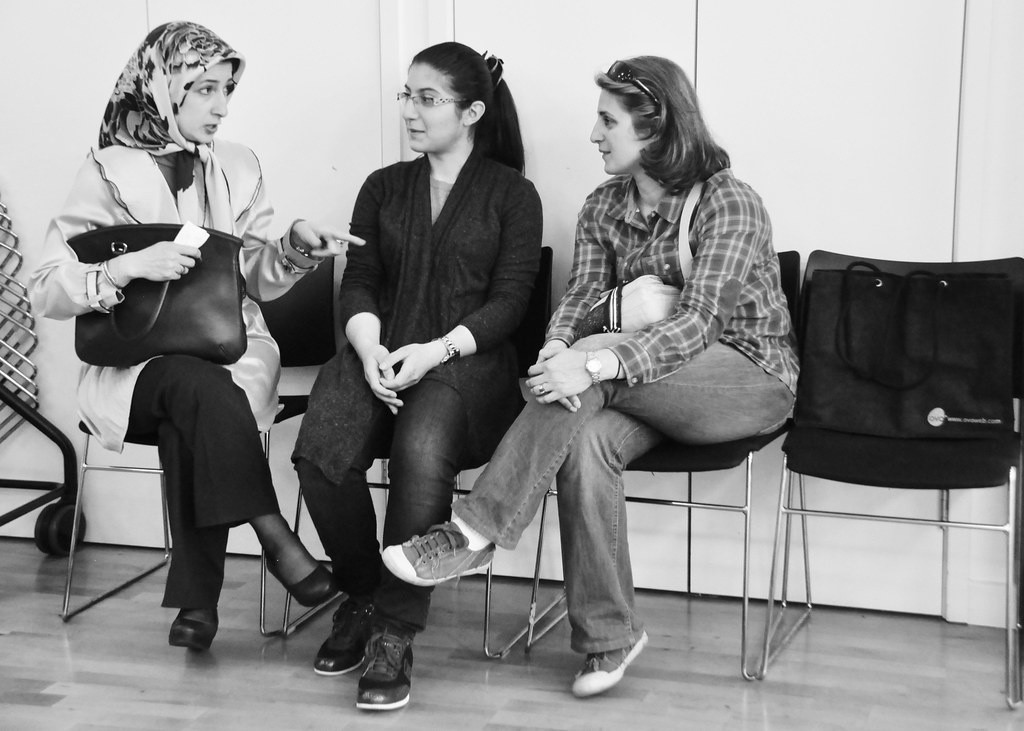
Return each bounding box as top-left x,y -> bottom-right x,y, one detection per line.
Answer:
754,248 -> 1024,710
275,247 -> 568,659
59,246 -> 339,637
521,251 -> 811,682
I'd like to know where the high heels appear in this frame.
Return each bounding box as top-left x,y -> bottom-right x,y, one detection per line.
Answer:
169,607 -> 218,650
266,532 -> 337,606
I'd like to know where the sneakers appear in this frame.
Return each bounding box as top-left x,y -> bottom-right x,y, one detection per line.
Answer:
382,521 -> 497,586
356,631 -> 413,710
573,630 -> 648,697
314,598 -> 371,676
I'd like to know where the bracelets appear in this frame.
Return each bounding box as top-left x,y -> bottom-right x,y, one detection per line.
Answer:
293,242 -> 310,257
440,336 -> 460,367
102,262 -> 124,291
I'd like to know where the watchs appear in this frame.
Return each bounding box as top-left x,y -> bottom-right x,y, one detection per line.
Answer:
586,352 -> 601,386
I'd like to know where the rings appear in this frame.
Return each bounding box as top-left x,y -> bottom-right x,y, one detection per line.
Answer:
178,265 -> 186,275
538,384 -> 546,394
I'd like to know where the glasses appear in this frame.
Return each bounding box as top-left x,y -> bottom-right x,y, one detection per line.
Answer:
397,92 -> 472,108
607,61 -> 660,107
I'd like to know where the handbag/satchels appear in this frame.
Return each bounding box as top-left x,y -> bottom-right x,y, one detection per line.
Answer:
66,223 -> 249,364
574,275 -> 680,336
795,262 -> 1015,438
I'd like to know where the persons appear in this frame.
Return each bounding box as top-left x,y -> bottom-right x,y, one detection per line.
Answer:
291,43 -> 544,708
382,56 -> 798,695
29,20 -> 366,650
